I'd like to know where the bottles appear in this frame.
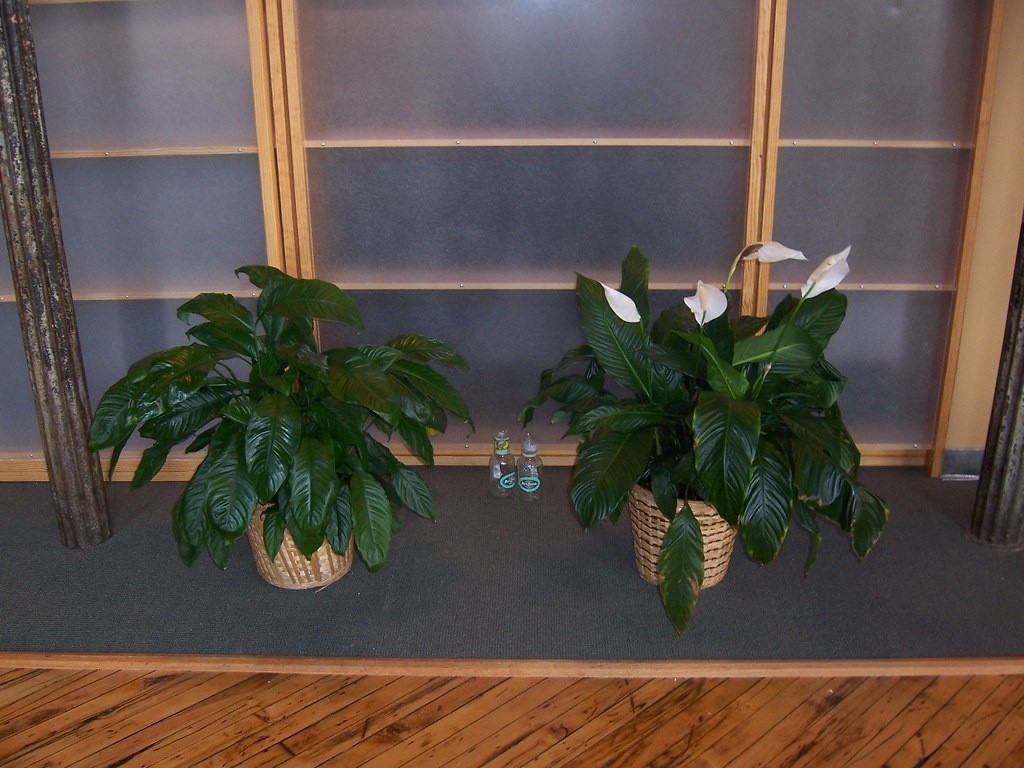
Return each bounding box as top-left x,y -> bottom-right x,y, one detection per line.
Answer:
489,430 -> 515,499
516,432 -> 544,501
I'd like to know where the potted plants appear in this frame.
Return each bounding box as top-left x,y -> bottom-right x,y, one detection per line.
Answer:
84,264 -> 475,589
518,239 -> 891,638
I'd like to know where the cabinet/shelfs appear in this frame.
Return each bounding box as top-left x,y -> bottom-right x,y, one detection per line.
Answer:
0,0 -> 1024,484
